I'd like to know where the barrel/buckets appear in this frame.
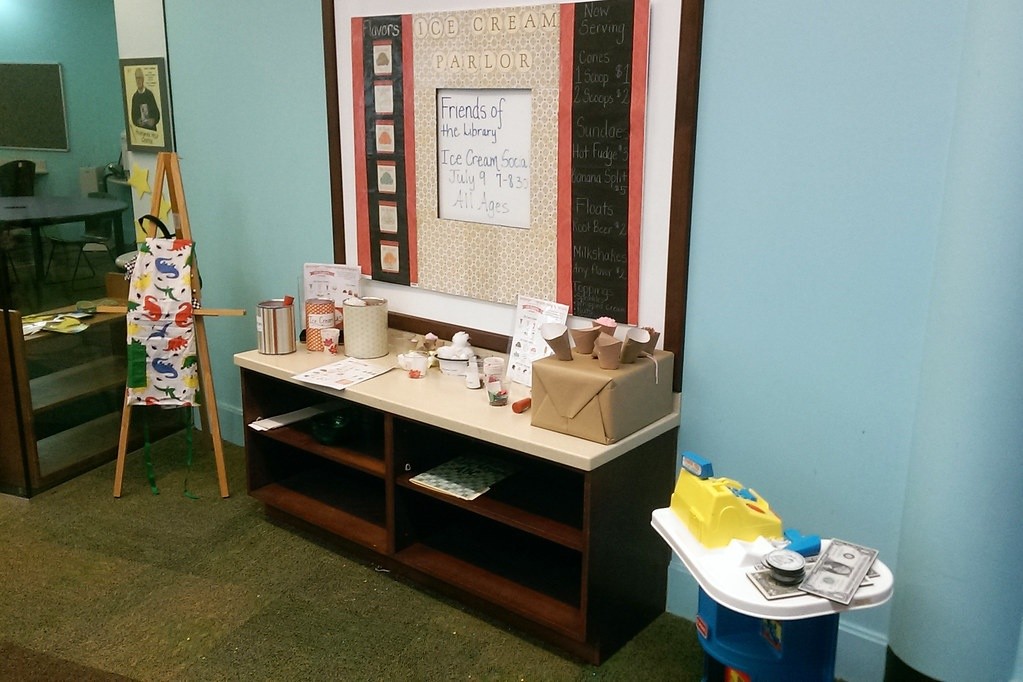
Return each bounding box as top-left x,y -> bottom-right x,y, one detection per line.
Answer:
343,297 -> 390,360
256,297 -> 298,356
305,297 -> 336,353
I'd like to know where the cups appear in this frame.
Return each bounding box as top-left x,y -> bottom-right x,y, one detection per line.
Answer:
321,329 -> 340,356
404,350 -> 429,379
487,375 -> 512,406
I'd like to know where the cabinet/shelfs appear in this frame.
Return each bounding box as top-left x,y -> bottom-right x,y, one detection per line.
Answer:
239,366 -> 681,670
1,272 -> 157,500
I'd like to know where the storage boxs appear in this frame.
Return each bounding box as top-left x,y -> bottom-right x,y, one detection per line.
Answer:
531,337 -> 675,445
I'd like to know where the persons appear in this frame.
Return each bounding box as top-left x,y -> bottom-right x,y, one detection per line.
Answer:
131,68 -> 160,130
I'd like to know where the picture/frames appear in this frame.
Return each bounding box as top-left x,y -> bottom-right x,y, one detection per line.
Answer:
119,57 -> 173,153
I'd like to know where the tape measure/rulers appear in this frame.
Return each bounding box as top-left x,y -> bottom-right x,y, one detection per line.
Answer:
75,300 -> 96,313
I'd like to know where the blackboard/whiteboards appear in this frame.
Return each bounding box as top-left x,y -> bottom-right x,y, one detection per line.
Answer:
435,88 -> 530,230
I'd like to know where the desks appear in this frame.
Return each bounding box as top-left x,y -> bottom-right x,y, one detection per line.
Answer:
0,195 -> 130,310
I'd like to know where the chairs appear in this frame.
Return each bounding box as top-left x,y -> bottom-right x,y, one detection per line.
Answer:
1,160 -> 36,288
45,191 -> 119,292
